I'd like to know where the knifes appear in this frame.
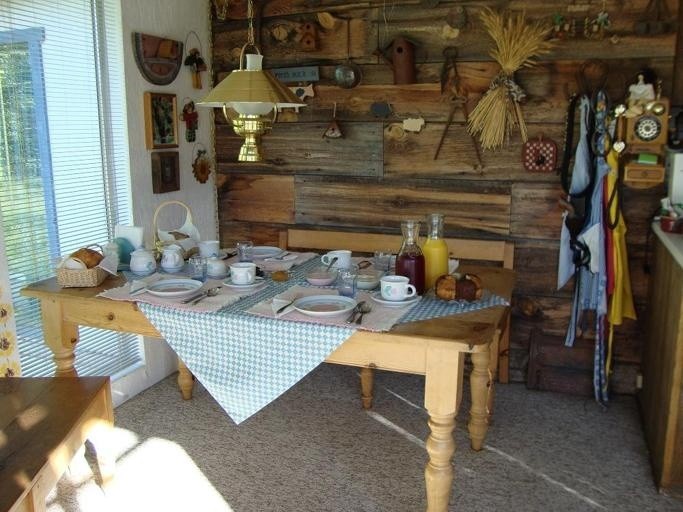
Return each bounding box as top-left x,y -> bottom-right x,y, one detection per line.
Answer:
181,286 -> 221,305
347,301 -> 367,324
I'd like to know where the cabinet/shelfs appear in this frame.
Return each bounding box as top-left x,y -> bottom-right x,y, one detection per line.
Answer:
638,218 -> 683,497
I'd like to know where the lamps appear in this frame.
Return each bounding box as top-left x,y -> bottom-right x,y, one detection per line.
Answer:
194,0 -> 309,163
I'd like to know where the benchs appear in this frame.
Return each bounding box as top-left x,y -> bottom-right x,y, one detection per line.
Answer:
270,175 -> 516,422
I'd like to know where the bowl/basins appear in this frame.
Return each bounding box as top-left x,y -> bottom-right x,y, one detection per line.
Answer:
334,64 -> 362,89
306,267 -> 336,286
351,275 -> 379,290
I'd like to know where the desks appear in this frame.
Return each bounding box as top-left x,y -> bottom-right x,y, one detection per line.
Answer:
0,375 -> 116,512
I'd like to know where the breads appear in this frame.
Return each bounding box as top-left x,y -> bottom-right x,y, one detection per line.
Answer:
435,273 -> 483,301
69,247 -> 103,269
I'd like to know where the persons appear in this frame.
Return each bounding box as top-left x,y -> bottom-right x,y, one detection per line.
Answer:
179,102 -> 199,143
155,102 -> 174,142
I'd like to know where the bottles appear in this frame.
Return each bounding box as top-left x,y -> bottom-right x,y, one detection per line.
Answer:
395,212 -> 449,296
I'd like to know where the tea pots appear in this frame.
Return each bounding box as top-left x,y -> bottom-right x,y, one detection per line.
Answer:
129,244 -> 185,277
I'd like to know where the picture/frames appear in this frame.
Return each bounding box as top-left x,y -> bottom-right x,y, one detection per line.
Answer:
130,31 -> 184,194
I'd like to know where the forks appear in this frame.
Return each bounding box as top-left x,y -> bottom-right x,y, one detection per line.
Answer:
355,306 -> 371,325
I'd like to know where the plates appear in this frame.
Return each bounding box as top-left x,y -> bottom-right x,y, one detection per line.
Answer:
370,292 -> 421,307
293,294 -> 357,319
144,279 -> 203,297
223,276 -> 265,289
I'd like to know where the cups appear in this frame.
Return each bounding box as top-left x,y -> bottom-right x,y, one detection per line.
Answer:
373,248 -> 391,271
189,240 -> 289,285
321,250 -> 358,298
379,275 -> 416,302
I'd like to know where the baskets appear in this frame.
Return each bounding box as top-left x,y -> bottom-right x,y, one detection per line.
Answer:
153,200 -> 198,260
53,244 -> 109,288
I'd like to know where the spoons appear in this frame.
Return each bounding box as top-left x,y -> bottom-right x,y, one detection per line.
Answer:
276,253 -> 291,260
191,287 -> 220,305
